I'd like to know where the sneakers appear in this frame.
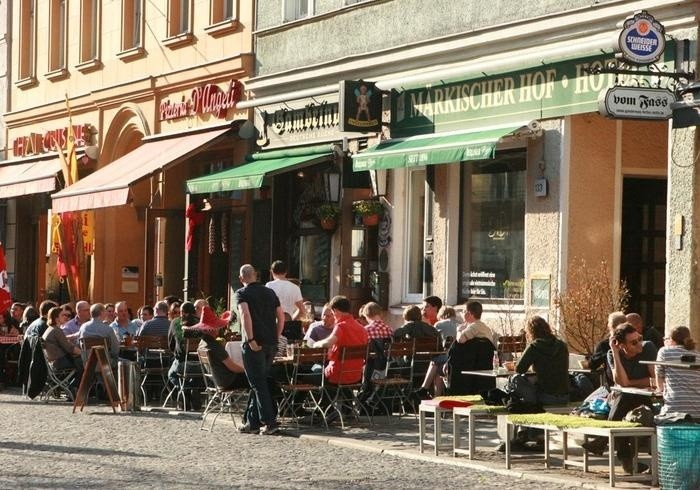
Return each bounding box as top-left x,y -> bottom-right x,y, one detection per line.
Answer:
260,424 -> 280,435
582,442 -> 605,456
402,388 -> 426,404
240,424 -> 260,434
630,462 -> 648,474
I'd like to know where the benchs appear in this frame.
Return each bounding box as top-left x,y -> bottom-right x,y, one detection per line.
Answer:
503,412 -> 658,488
413,394 -> 485,457
450,402 -> 582,461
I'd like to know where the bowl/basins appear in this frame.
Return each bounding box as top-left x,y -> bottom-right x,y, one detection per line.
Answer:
578,360 -> 589,370
502,362 -> 514,371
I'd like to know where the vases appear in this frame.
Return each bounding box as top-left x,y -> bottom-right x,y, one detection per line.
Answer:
362,214 -> 378,226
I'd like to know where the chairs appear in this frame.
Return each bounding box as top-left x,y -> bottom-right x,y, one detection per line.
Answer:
1,331 -> 528,439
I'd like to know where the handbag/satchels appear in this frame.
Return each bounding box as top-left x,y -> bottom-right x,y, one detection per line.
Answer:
570,370 -> 600,401
626,404 -> 653,426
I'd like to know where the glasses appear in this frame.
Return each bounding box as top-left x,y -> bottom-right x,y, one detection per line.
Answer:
632,335 -> 644,345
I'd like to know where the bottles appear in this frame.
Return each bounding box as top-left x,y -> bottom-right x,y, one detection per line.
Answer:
649,377 -> 656,391
287,343 -> 309,360
492,350 -> 500,374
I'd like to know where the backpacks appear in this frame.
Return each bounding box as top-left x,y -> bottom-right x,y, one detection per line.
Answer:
505,373 -> 545,413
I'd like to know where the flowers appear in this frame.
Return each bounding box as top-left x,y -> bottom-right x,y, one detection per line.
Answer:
353,199 -> 385,216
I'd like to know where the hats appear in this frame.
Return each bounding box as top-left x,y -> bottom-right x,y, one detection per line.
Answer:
479,388 -> 505,404
181,307 -> 230,331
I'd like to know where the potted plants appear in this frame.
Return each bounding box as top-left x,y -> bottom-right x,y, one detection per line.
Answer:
315,203 -> 341,232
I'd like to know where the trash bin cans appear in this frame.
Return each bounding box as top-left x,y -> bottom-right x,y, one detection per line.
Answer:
655,421 -> 700,490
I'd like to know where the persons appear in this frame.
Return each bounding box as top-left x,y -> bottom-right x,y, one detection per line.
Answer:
355,85 -> 371,122
235,263 -> 287,436
0,296 -> 250,413
282,294 -> 495,425
497,310 -> 700,481
262,259 -> 305,323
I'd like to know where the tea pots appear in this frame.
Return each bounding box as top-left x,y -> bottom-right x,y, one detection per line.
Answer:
123,332 -> 133,346
